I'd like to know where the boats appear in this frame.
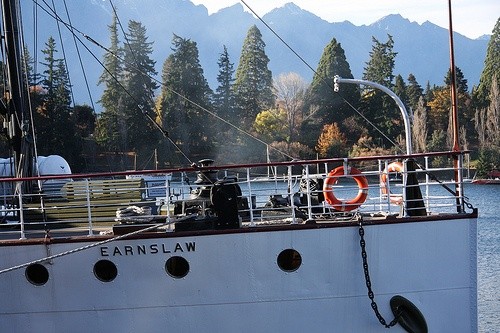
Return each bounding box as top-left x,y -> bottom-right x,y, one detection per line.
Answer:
0,0 -> 481,333
124,149 -> 173,197
470,167 -> 500,186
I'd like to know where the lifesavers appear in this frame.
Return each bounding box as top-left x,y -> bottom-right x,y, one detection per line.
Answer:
323,165 -> 369,211
381,161 -> 404,205
